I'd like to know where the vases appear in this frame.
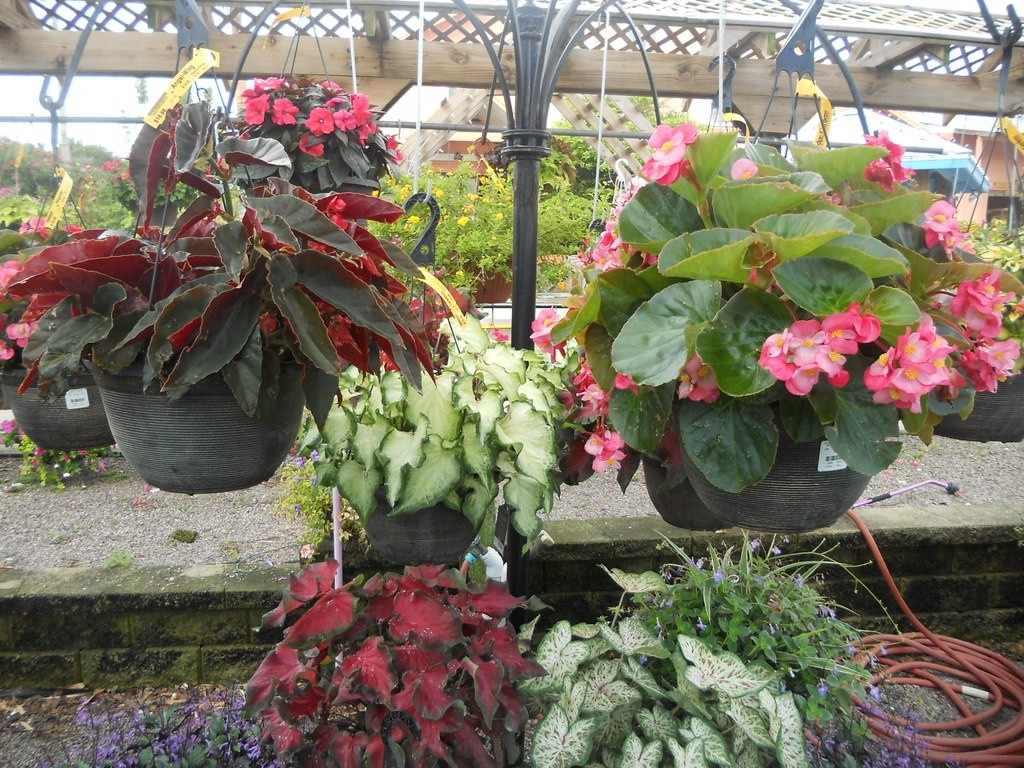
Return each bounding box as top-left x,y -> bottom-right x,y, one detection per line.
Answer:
290,177 -> 379,230
2,369 -> 116,450
933,371 -> 1024,444
449,253 -> 513,303
642,457 -> 734,531
673,401 -> 872,532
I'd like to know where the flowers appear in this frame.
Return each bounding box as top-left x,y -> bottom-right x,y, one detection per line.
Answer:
530,122 -> 1024,494
366,146 -> 584,293
240,78 -> 406,190
0,217 -> 84,372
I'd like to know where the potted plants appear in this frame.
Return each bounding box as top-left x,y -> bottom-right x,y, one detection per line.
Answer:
299,315 -> 568,568
6,100 -> 437,496
